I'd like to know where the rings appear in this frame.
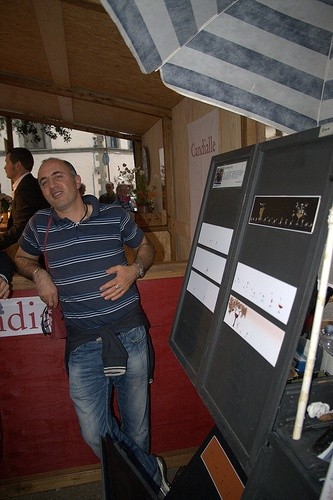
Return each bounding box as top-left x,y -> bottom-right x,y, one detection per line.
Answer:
115,284 -> 119,289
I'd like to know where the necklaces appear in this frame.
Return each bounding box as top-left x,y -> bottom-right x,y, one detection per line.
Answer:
80,203 -> 88,222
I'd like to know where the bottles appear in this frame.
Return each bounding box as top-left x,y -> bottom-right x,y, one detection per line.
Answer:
319,325 -> 333,356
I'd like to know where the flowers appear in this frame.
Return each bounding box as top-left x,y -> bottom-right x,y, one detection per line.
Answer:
117,159 -> 158,203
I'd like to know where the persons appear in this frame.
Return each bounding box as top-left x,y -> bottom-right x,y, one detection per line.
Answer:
0,147 -> 51,300
13,157 -> 155,500
79,182 -> 134,212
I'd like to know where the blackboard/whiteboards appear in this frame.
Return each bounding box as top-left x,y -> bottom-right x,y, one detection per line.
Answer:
168,127 -> 332,476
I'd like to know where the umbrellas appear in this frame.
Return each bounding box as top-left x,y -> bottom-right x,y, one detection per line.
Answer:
98,0 -> 333,440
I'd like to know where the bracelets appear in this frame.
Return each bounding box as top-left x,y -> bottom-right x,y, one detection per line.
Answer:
29,264 -> 41,275
133,262 -> 146,278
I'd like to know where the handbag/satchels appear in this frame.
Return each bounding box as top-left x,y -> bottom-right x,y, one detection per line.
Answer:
42,297 -> 67,339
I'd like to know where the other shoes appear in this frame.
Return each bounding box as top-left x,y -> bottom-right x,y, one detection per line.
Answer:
155,457 -> 170,496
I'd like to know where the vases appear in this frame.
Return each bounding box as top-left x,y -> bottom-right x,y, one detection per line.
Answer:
137,204 -> 152,213
